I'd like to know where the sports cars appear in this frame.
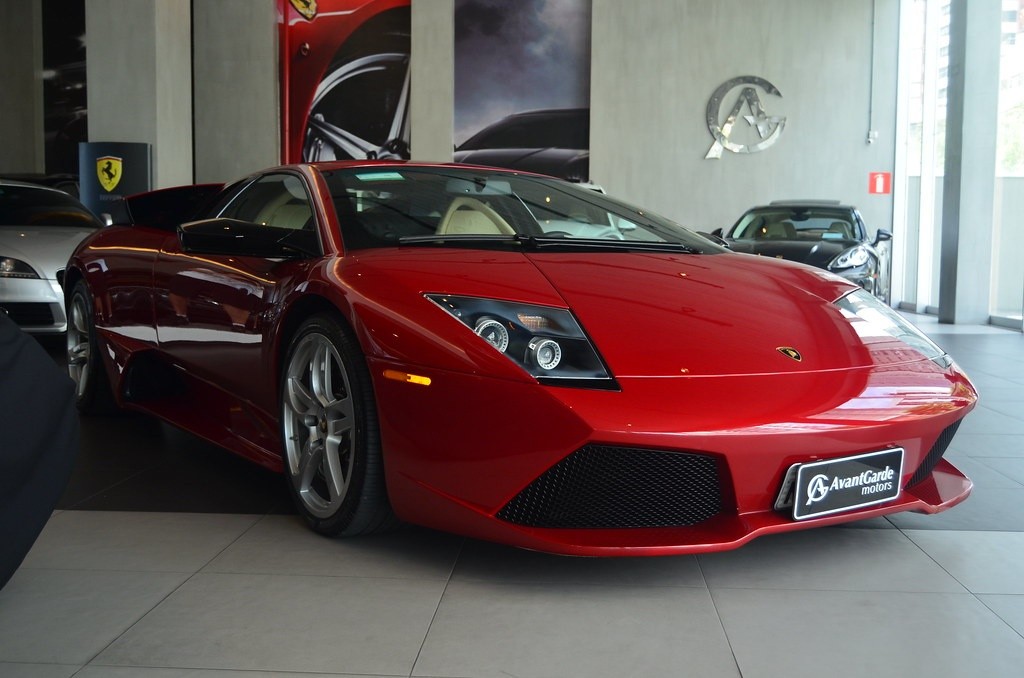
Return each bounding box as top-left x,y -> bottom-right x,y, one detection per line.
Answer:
62,157 -> 978,558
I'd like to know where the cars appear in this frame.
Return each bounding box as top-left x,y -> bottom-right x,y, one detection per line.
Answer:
345,171 -> 405,214
712,198 -> 893,298
452,107 -> 590,182
43,58 -> 87,141
300,47 -> 414,165
537,184 -> 636,242
0,180 -> 114,337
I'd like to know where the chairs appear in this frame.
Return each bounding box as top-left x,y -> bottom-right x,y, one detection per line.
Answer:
828,221 -> 854,241
761,222 -> 792,240
435,197 -> 516,236
255,192 -> 311,229
